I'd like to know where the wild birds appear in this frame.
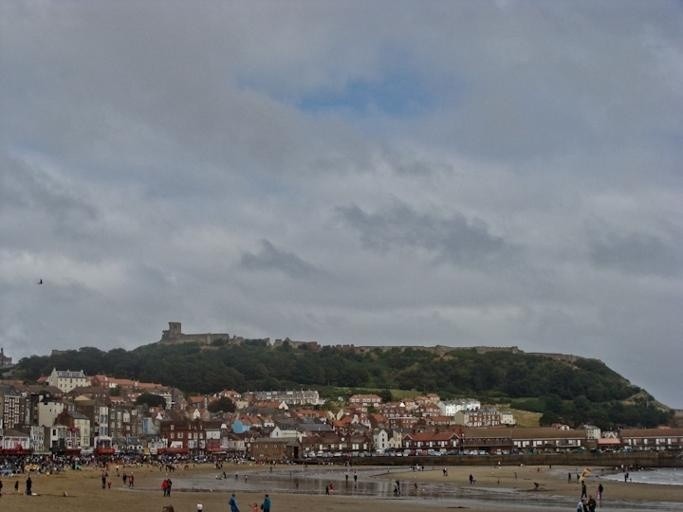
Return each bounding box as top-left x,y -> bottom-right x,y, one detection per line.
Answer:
37,278 -> 42,284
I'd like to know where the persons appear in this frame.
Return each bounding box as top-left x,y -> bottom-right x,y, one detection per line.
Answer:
101,468 -> 112,489
123,469 -> 136,488
25,477 -> 33,495
161,505 -> 174,512
577,480 -> 603,512
161,479 -> 172,498
228,493 -> 271,512
196,504 -> 203,512
